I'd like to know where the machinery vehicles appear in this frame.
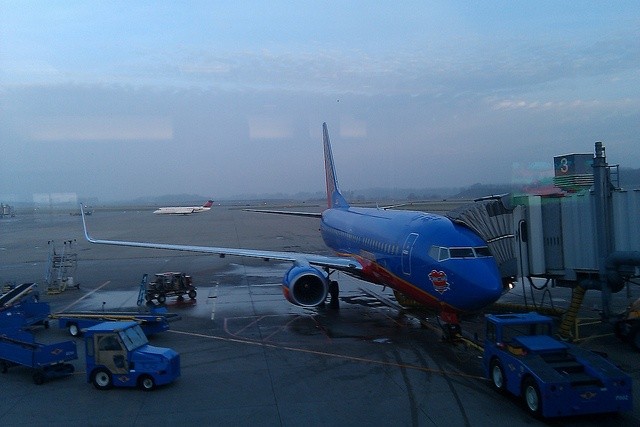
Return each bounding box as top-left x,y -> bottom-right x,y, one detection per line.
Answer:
145,272 -> 197,303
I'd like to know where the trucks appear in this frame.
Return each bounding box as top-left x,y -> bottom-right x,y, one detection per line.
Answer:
0,282 -> 51,331
0,321 -> 181,391
483,312 -> 633,418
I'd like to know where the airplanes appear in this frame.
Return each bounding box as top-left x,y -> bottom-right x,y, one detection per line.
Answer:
153,200 -> 214,215
80,123 -> 504,338
